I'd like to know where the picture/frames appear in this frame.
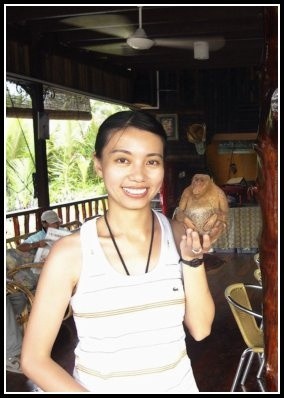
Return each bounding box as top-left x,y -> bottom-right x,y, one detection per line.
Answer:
156,114 -> 178,140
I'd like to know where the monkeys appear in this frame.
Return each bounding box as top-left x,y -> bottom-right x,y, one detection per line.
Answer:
176,172 -> 227,243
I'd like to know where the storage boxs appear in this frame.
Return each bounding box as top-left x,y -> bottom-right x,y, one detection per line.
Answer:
226,177 -> 248,185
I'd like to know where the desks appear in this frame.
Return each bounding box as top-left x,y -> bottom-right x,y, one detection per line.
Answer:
209,204 -> 261,248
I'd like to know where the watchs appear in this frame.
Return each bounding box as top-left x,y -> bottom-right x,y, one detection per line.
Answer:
178,257 -> 204,268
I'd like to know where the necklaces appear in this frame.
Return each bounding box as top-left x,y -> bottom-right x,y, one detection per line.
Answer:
105,208 -> 155,274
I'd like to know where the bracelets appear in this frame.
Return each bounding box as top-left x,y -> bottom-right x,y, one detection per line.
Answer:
30,243 -> 33,250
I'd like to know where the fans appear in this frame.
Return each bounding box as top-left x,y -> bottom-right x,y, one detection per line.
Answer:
63,5 -> 227,57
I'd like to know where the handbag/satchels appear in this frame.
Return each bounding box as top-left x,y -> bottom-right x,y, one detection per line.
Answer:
6,289 -> 31,370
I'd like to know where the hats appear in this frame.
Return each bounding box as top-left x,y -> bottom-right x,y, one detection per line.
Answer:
41,211 -> 62,224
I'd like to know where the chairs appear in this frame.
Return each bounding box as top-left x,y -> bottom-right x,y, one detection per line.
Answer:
224,283 -> 263,392
6,246 -> 73,393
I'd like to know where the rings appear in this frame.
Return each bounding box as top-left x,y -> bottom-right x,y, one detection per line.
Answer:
192,248 -> 202,254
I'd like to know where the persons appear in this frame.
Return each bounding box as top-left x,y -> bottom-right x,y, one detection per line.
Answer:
20,110 -> 215,394
17,210 -> 68,286
